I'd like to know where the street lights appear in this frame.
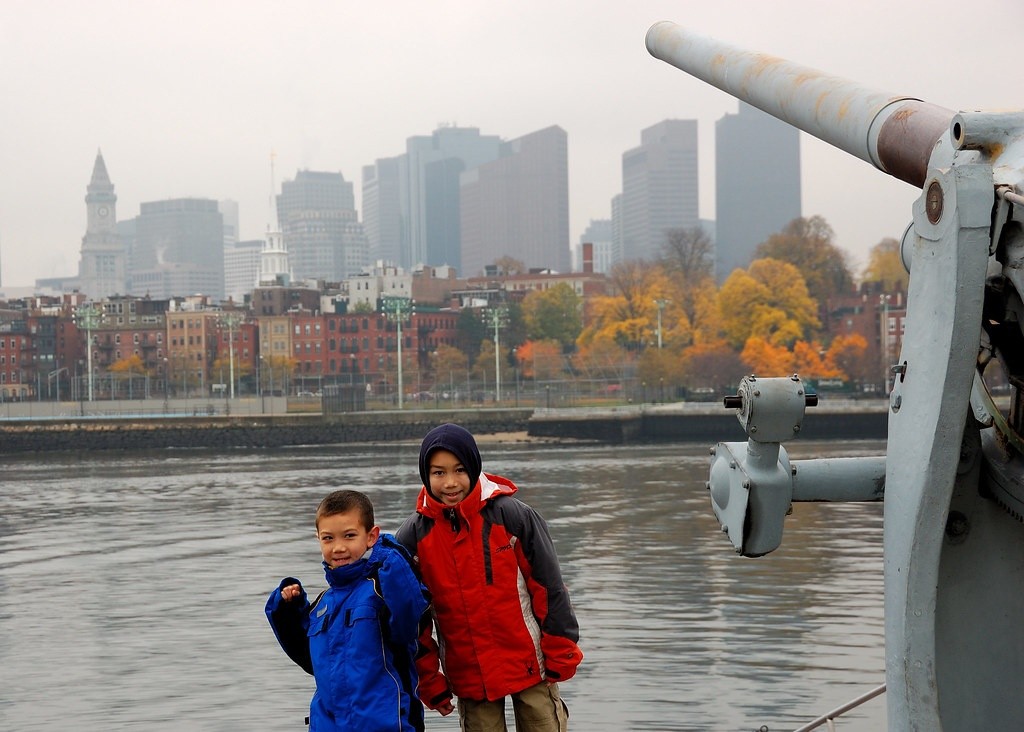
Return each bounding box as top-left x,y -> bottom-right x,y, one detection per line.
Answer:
481,307 -> 511,403
434,351 -> 443,406
216,312 -> 241,412
71,307 -> 102,404
163,358 -> 169,406
653,299 -> 669,349
513,348 -> 520,406
350,354 -> 357,387
381,298 -> 416,409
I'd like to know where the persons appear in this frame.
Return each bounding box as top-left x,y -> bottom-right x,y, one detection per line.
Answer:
263,490 -> 433,732
393,423 -> 583,732
